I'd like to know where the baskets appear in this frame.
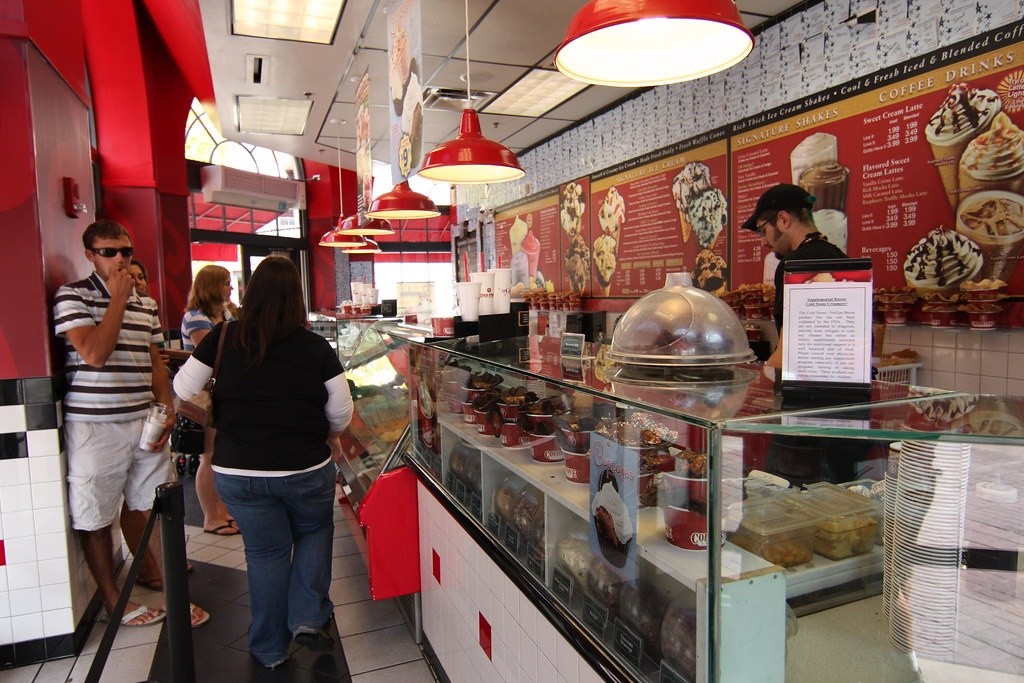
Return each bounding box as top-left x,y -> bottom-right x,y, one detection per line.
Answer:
878,363 -> 922,400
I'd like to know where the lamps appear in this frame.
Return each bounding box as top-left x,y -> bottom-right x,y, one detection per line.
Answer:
318,118 -> 369,247
340,236 -> 383,254
336,214 -> 396,236
417,0 -> 525,186
363,180 -> 441,220
554,0 -> 754,90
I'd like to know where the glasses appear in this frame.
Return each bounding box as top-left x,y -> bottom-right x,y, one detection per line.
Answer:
91,246 -> 133,257
757,211 -> 779,237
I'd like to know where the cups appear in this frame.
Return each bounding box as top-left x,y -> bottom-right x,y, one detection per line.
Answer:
470,272 -> 495,316
361,283 -> 372,304
487,268 -> 512,314
369,288 -> 379,303
350,281 -> 363,304
456,281 -> 482,321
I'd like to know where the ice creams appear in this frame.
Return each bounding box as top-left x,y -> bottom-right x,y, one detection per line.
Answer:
560,182 -> 625,295
901,82 -> 1024,300
671,160 -> 728,297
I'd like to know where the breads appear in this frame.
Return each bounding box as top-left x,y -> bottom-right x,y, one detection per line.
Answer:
348,380 -> 411,443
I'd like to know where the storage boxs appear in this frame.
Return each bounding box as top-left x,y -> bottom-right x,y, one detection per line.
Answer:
725,492 -> 826,568
786,481 -> 883,560
872,356 -> 923,385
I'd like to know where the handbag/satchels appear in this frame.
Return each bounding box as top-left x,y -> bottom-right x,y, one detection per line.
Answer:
176,378 -> 216,427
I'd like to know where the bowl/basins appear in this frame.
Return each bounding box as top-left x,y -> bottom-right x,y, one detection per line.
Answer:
445,378 -> 972,662
529,291 -> 582,311
340,305 -> 372,315
405,314 -> 418,324
431,317 -> 454,336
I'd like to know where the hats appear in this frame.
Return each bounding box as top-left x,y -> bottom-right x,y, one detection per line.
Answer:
741,183 -> 816,231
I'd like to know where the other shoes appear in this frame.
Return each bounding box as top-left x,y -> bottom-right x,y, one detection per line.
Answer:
294,616 -> 335,650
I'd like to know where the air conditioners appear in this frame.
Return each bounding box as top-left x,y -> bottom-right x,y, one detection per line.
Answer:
200,165 -> 301,213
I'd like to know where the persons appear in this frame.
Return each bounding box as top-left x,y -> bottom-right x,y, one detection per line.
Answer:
53,219 -> 210,629
742,182 -> 874,491
173,257 -> 354,670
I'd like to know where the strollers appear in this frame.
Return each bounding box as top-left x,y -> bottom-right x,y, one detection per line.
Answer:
172,410 -> 206,477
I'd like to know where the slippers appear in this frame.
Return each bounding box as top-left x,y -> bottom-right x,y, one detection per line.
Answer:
98,605 -> 167,627
186,564 -> 194,574
204,519 -> 241,535
160,602 -> 210,627
135,579 -> 163,591
